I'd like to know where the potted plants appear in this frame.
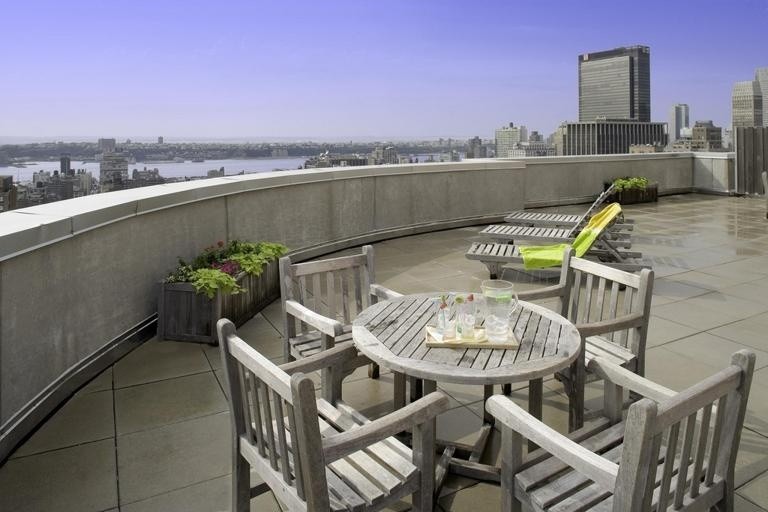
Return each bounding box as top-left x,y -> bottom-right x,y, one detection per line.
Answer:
605,177 -> 659,205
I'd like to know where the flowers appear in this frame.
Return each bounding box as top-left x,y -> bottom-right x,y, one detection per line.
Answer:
166,238 -> 290,298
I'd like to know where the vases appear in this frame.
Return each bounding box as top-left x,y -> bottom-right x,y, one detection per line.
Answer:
157,258 -> 279,344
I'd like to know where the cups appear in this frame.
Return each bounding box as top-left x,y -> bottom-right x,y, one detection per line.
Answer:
436,280 -> 518,343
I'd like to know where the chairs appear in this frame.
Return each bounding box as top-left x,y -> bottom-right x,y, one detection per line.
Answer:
478,189 -> 632,250
465,202 -> 653,291
277,243 -> 406,405
217,317 -> 450,510
485,348 -> 754,510
505,185 -> 636,233
502,245 -> 655,435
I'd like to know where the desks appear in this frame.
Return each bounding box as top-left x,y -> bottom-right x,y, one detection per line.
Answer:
351,291 -> 582,504
